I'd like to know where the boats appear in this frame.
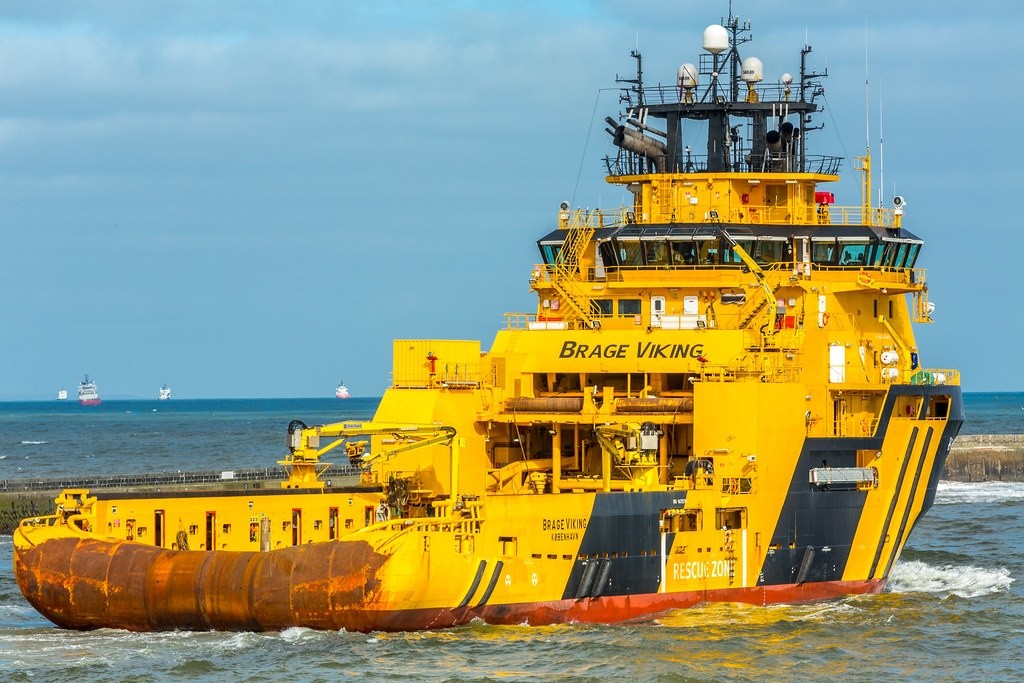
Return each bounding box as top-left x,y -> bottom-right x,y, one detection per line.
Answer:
8,1 -> 965,635
76,374 -> 103,406
157,383 -> 173,400
336,380 -> 351,399
57,387 -> 69,400
